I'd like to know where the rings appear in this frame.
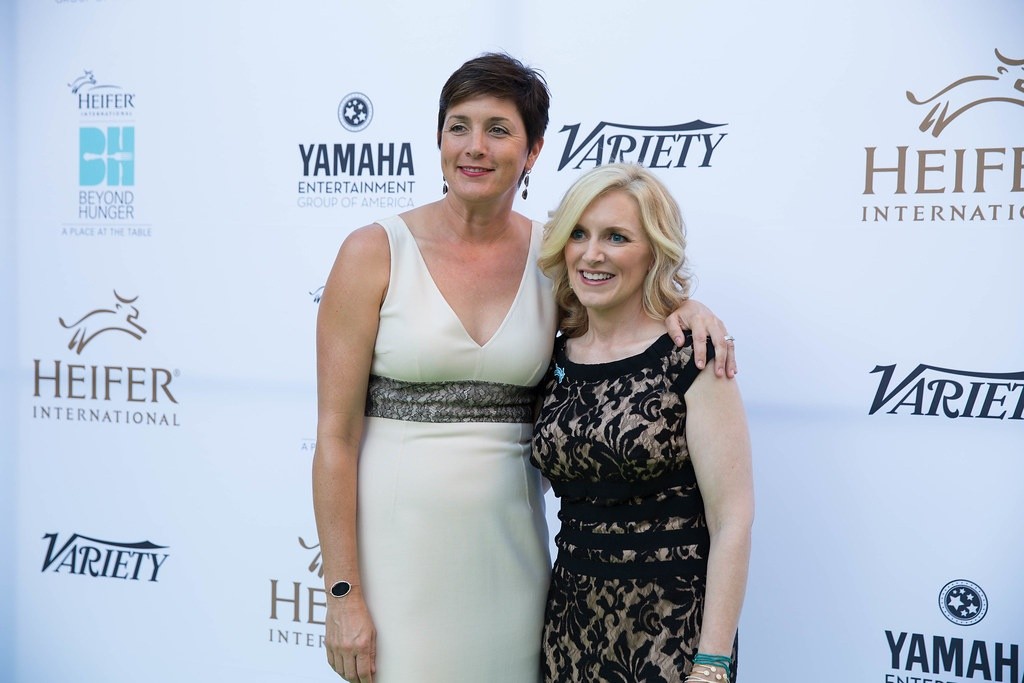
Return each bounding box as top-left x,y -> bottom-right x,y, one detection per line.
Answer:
723,335 -> 734,341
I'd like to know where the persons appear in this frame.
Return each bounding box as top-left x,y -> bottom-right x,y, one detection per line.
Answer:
530,162 -> 758,683
307,54 -> 740,682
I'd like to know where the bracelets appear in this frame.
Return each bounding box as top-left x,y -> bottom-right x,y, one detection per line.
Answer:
325,581 -> 362,598
684,653 -> 732,683
